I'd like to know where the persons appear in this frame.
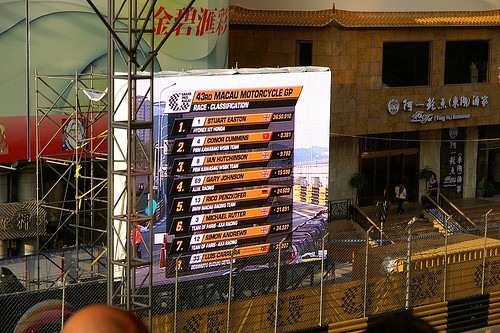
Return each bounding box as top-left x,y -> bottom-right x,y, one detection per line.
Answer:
144,192 -> 157,228
376,188 -> 391,224
58,304 -> 150,333
395,180 -> 406,214
291,243 -> 298,260
428,174 -> 438,202
130,223 -> 143,259
